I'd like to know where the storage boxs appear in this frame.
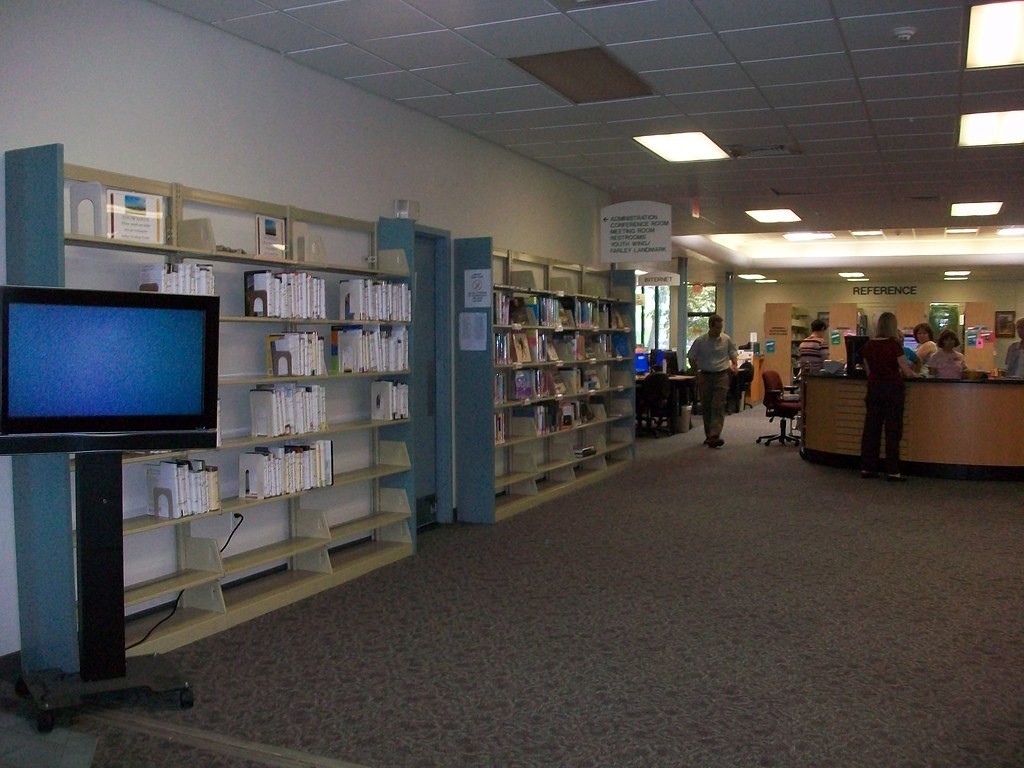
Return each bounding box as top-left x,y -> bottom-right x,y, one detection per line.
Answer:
672,405 -> 692,433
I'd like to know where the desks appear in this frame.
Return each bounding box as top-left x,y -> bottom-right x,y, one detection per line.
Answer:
635,373 -> 696,430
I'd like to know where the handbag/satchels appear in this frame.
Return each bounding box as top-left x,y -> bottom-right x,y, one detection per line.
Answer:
824,359 -> 844,374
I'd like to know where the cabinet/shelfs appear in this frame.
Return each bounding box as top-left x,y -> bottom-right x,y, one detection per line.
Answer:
744,355 -> 763,403
829,303 -> 867,362
762,303 -> 810,400
454,237 -> 636,525
4,143 -> 419,714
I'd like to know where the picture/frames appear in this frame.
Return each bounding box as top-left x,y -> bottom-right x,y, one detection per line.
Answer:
995,310 -> 1016,339
818,312 -> 829,328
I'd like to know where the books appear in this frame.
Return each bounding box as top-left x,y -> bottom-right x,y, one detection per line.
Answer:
265,325 -> 410,378
249,374 -> 410,436
494,288 -> 630,459
144,439 -> 335,519
142,263 -> 413,321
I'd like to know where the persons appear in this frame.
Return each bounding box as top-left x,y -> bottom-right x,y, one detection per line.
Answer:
1004,317 -> 1024,379
913,322 -> 938,370
797,318 -> 830,377
685,315 -> 740,447
928,330 -> 969,380
857,312 -> 926,483
897,329 -> 922,373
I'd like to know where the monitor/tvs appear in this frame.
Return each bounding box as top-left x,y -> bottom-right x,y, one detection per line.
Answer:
903,334 -> 919,350
844,335 -> 870,377
0,285 -> 218,455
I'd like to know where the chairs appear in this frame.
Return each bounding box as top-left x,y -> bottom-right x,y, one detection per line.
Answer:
726,362 -> 754,413
756,370 -> 801,448
636,373 -> 672,439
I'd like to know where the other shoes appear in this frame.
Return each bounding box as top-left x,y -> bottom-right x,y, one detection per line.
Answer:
860,468 -> 882,479
887,471 -> 908,480
704,435 -> 724,448
